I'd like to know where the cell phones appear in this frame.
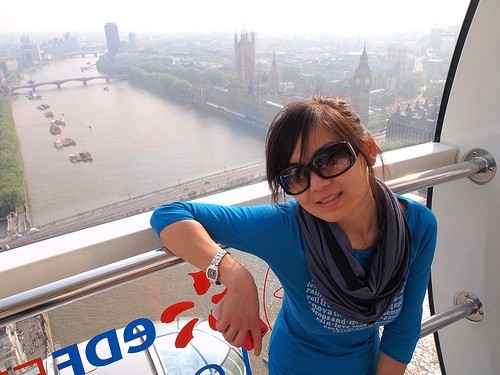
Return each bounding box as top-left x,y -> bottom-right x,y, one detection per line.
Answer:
212,293 -> 269,352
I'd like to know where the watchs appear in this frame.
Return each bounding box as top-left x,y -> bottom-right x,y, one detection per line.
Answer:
206,249 -> 231,286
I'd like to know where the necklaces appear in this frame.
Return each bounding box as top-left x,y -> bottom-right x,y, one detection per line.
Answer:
362,214 -> 378,250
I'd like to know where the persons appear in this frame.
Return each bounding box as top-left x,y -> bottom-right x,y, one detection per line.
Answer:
150,97 -> 438,375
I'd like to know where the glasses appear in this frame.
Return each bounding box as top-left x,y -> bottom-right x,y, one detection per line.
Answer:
279,141 -> 356,195
